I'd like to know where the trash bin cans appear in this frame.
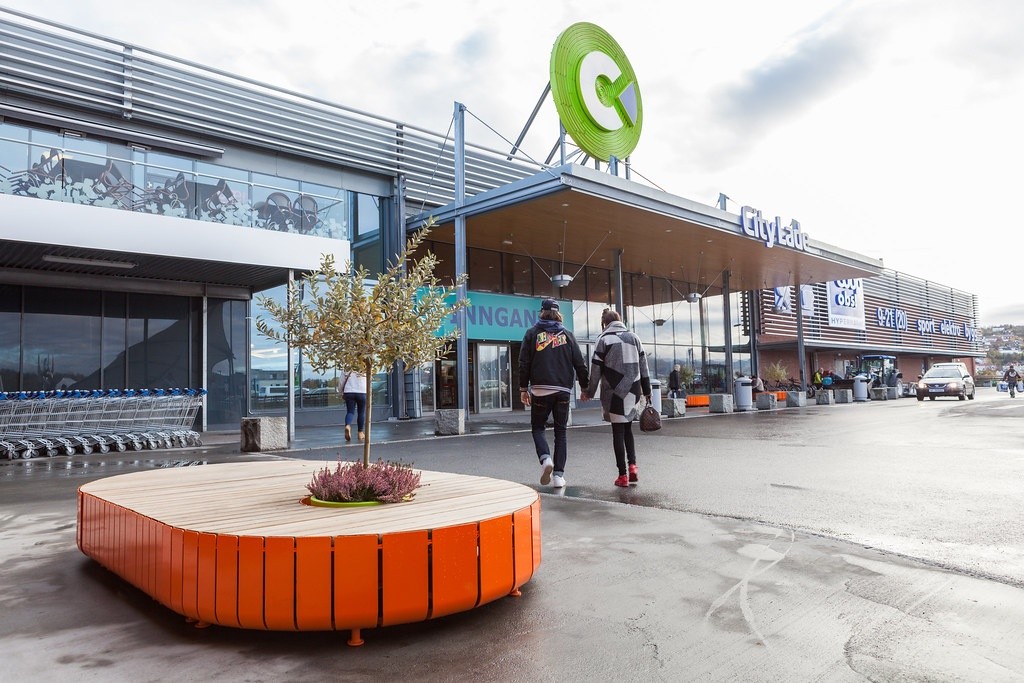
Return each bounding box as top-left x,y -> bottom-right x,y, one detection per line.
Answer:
735,377 -> 753,410
641,379 -> 662,416
853,375 -> 868,401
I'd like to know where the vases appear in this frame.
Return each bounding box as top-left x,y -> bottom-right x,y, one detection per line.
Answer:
311,494 -> 410,508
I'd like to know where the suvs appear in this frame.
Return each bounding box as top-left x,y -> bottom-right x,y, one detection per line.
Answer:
480,378 -> 508,407
370,381 -> 389,404
689,378 -> 705,389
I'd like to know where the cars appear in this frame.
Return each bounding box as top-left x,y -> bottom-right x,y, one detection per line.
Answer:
916,367 -> 976,402
281,388 -> 344,405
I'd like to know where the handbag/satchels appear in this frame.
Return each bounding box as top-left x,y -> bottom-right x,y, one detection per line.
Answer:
640,398 -> 660,431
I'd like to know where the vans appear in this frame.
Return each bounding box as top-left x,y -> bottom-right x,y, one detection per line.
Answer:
258,386 -> 300,403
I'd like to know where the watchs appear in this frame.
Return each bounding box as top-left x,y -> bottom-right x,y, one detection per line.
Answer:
519,387 -> 528,392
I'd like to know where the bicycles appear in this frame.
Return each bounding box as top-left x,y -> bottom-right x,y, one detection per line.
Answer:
761,377 -> 817,399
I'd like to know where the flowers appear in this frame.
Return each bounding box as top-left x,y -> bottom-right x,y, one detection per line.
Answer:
304,454 -> 431,503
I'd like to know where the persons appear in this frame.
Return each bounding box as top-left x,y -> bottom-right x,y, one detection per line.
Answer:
812,365 -> 880,398
582,308 -> 653,487
1002,365 -> 1023,399
751,375 -> 764,402
735,371 -> 744,379
667,364 -> 681,398
518,299 -> 591,488
701,372 -> 723,394
338,352 -> 367,442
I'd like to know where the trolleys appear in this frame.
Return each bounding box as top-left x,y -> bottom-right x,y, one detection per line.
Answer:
0,387 -> 208,461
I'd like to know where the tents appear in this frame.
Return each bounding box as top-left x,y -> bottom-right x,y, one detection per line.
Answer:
66,298 -> 237,410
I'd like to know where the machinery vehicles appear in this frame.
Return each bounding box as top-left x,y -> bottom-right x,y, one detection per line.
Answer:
849,354 -> 905,399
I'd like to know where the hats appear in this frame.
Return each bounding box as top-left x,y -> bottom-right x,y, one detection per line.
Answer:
537,298 -> 560,312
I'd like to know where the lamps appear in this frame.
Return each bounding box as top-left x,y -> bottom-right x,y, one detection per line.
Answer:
511,221 -> 611,288
741,270 -> 813,315
649,251 -> 734,303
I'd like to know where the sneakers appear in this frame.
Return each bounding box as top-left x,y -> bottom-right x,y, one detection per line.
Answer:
358,432 -> 365,439
553,475 -> 566,488
615,475 -> 628,487
344,425 -> 351,440
539,458 -> 554,486
628,465 -> 639,481
